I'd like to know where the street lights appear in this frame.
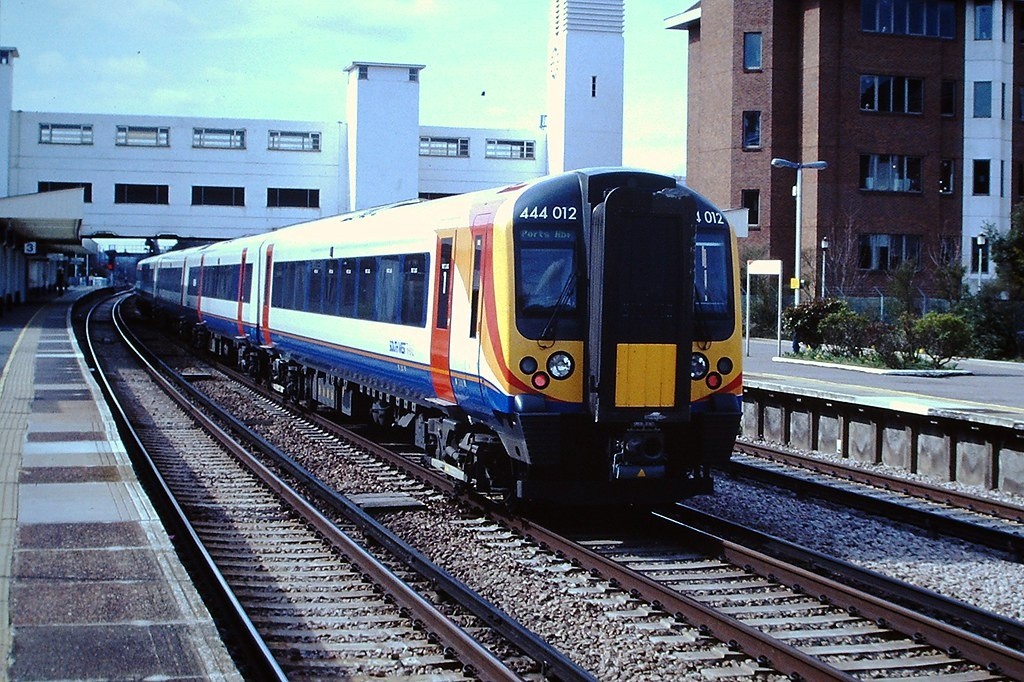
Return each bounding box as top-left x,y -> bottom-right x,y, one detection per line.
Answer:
819,234 -> 828,299
976,233 -> 986,294
771,156 -> 828,307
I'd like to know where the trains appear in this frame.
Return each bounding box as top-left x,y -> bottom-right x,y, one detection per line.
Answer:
135,166 -> 748,534
